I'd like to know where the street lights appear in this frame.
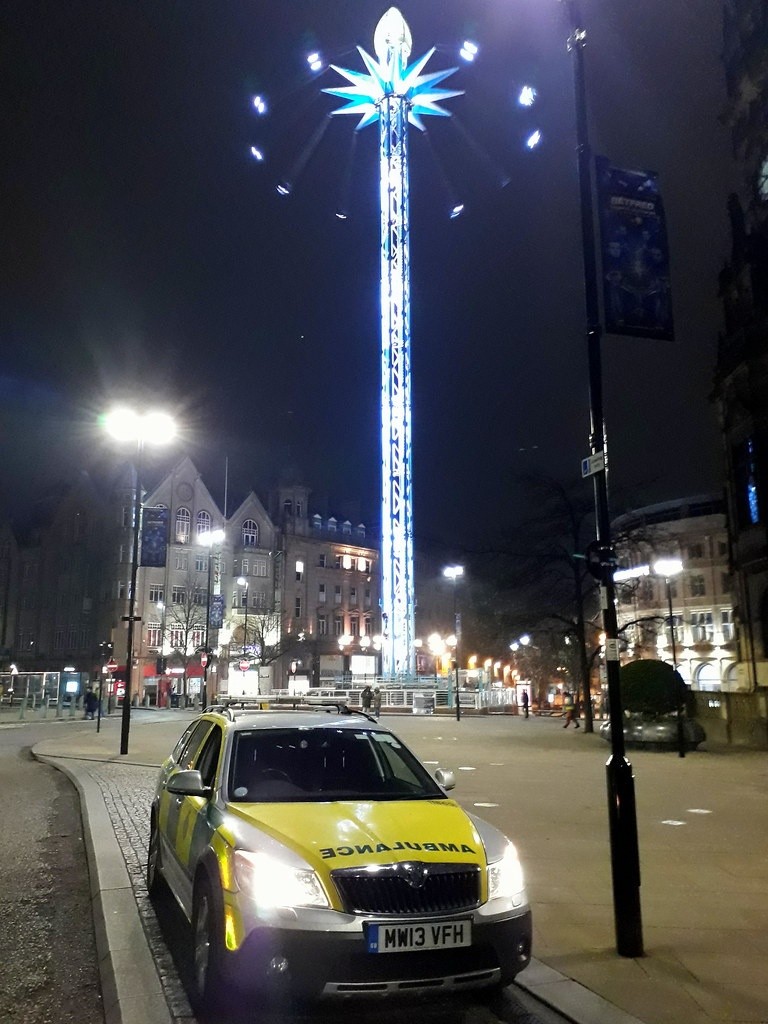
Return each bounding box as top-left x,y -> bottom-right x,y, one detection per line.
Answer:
442,564 -> 464,721
157,601 -> 167,709
198,528 -> 225,714
96,640 -> 115,733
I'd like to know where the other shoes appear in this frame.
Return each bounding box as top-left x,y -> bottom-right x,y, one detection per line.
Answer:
564,726 -> 566,728
575,726 -> 578,728
525,716 -> 528,718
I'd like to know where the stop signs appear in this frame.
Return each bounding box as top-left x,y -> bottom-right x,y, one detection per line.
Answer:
239,659 -> 250,674
200,652 -> 209,667
106,657 -> 118,673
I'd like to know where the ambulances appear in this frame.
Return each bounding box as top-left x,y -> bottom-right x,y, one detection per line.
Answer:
146,692 -> 533,1010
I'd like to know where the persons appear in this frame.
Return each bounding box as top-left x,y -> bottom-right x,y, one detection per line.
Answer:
361,686 -> 381,719
83,686 -> 106,720
523,693 -> 529,718
563,691 -> 580,729
